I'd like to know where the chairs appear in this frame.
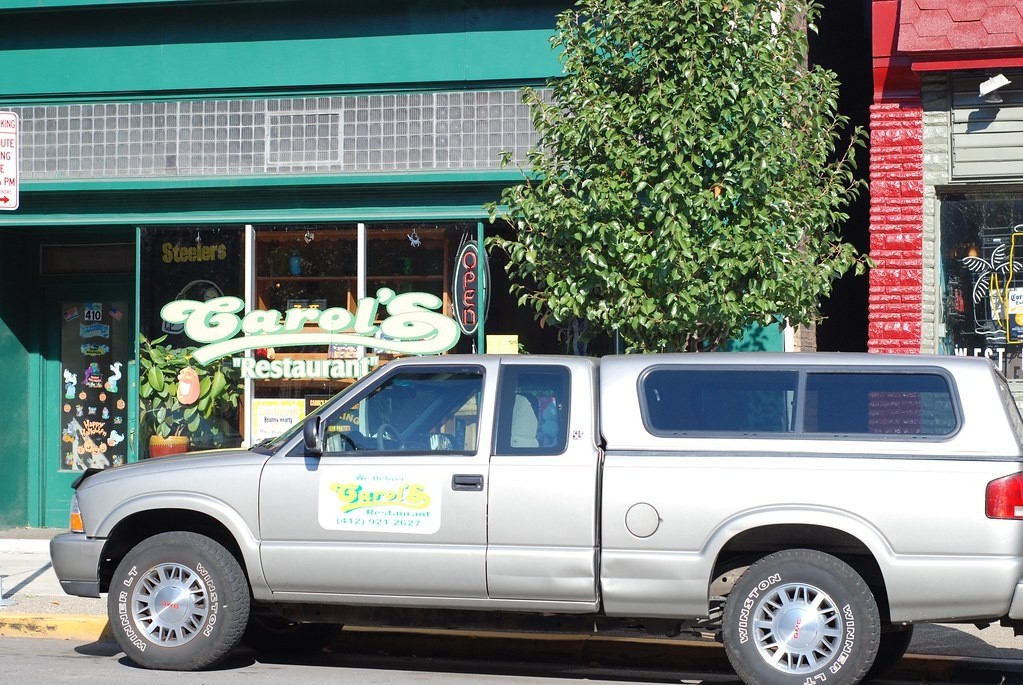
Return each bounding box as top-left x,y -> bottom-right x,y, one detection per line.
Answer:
510,393 -> 558,448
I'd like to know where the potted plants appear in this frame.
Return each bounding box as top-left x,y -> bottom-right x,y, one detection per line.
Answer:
134,332 -> 245,458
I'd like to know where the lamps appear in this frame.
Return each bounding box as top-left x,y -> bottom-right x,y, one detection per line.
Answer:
978,73 -> 1012,104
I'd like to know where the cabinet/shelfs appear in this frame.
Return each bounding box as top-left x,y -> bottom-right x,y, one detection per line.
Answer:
240,229 -> 452,439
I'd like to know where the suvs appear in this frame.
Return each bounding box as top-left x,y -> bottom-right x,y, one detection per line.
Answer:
50,354 -> 1023,685
